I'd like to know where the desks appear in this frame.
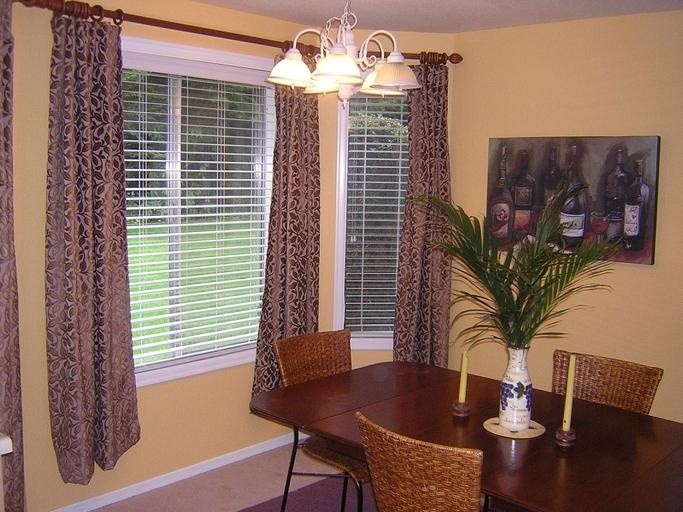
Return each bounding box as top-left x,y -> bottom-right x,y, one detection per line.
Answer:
249,362 -> 682,512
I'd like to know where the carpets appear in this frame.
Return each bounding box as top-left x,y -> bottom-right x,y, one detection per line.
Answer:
241,472 -> 377,512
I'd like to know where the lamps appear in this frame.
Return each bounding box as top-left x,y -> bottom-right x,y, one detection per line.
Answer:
266,0 -> 422,108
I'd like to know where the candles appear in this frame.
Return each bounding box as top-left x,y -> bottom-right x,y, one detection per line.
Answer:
562,355 -> 574,432
457,351 -> 468,404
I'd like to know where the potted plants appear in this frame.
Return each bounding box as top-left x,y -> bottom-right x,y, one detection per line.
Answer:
406,161 -> 627,432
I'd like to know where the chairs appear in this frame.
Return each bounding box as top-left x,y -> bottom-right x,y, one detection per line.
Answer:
355,410 -> 489,511
272,330 -> 371,512
552,350 -> 663,414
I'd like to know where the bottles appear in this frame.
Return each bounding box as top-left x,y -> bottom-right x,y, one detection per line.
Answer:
492,144 -> 650,252
